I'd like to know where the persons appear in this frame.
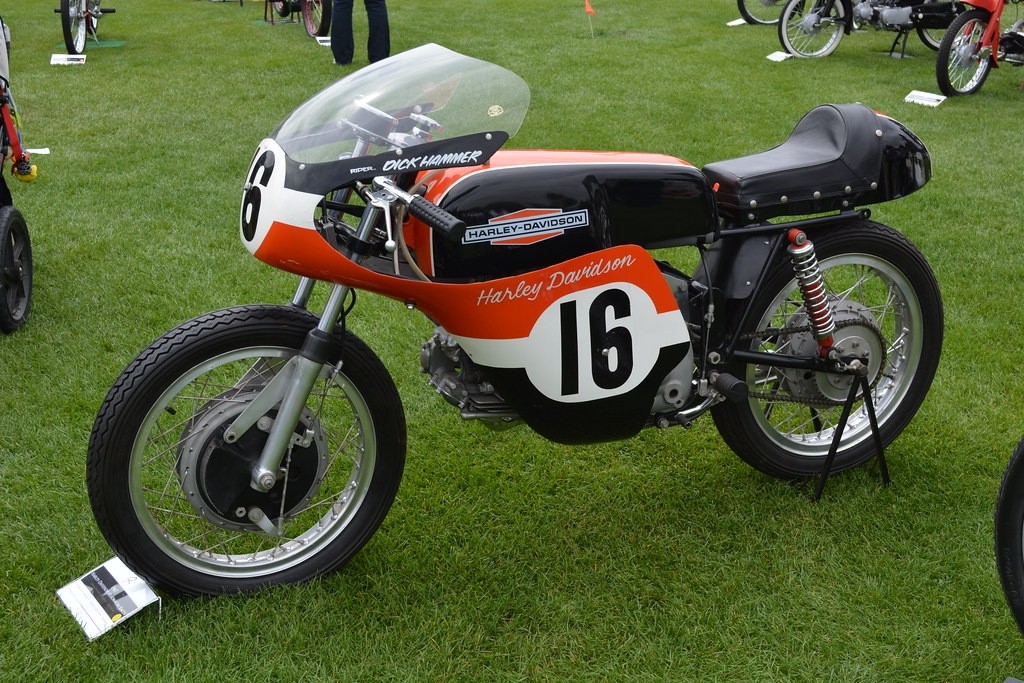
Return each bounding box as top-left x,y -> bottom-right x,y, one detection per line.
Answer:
329,0 -> 390,67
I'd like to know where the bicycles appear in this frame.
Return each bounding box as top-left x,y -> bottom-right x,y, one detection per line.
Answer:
53,0 -> 115,55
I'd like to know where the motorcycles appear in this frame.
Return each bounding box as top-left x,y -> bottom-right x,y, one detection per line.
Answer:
86,42 -> 946,604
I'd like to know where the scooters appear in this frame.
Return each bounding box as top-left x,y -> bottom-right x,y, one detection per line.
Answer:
737,0 -> 1024,97
0,76 -> 38,334
270,0 -> 333,38
